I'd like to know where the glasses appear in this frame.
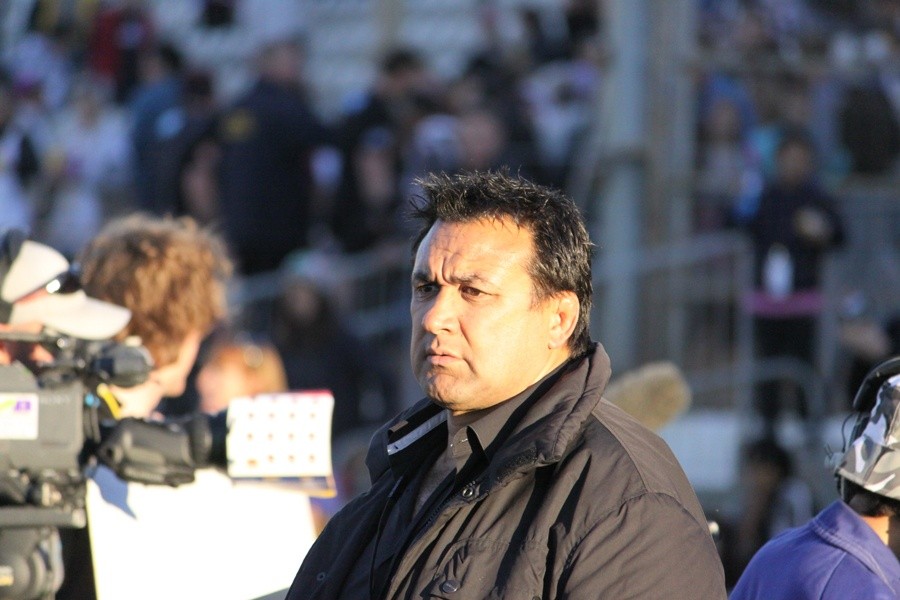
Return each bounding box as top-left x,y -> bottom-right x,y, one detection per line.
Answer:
12,270 -> 83,305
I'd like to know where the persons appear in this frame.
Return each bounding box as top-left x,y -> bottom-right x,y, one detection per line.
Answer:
0,0 -> 900,591
728,355 -> 900,600
284,168 -> 731,600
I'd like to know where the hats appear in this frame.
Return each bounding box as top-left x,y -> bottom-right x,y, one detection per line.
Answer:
836,374 -> 900,500
852,357 -> 900,412
2,238 -> 132,341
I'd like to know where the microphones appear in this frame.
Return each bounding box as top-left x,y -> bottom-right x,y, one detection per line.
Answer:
98,349 -> 150,388
